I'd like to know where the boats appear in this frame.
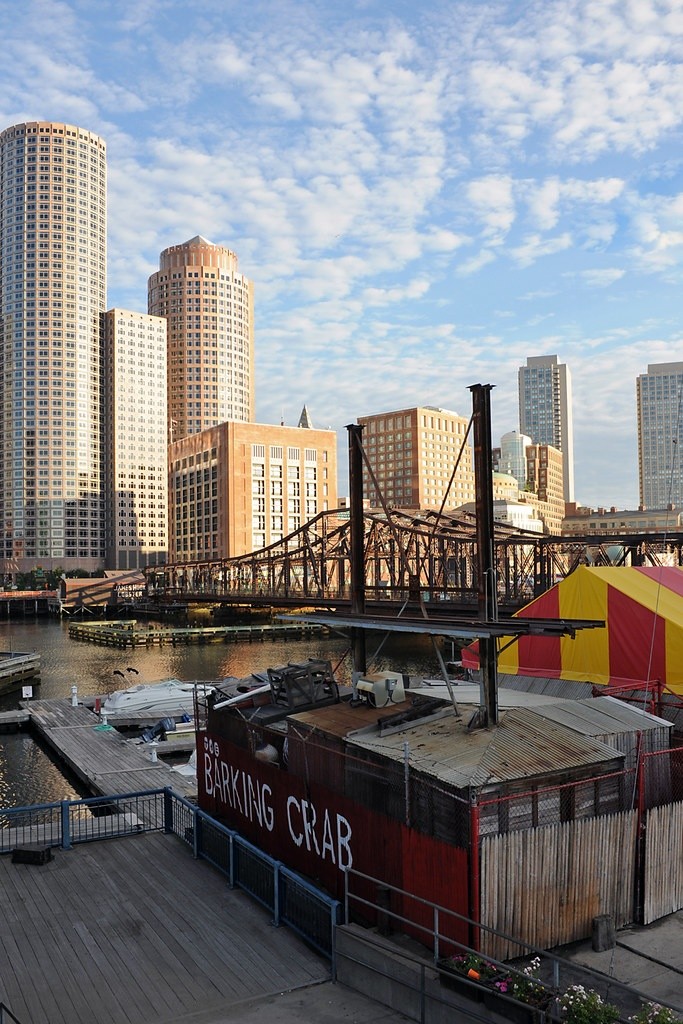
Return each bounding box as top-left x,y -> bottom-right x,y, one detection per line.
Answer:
129,717 -> 199,755
98,676 -> 214,726
